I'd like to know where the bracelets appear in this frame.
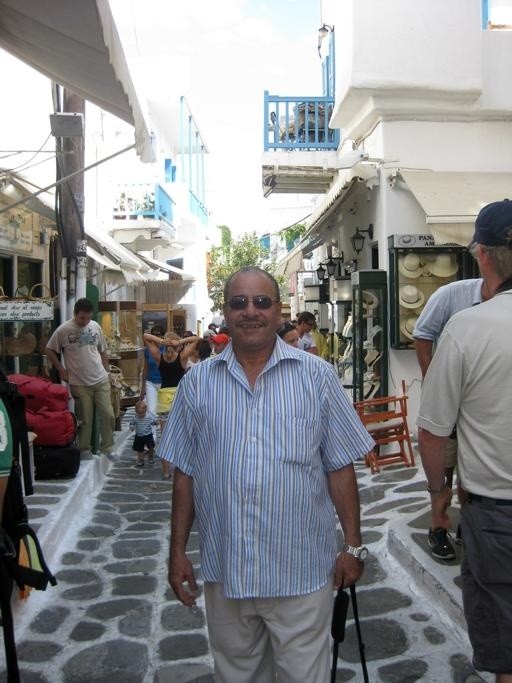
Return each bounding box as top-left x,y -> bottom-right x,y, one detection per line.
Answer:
426,476 -> 449,496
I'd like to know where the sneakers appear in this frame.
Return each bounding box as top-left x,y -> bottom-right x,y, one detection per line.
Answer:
162,471 -> 172,481
136,455 -> 156,466
426,525 -> 456,561
455,523 -> 464,545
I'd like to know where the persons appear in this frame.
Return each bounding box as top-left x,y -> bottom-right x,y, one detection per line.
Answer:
415,200 -> 511,683
154,266 -> 377,682
0,399 -> 13,511
411,278 -> 491,559
46,298 -> 119,463
129,312 -> 332,481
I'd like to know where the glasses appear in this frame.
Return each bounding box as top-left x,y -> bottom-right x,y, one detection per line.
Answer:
224,295 -> 280,311
303,320 -> 314,326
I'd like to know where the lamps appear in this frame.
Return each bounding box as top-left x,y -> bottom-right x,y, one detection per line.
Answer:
315,263 -> 327,281
0,174 -> 15,197
349,224 -> 373,255
303,277 -> 329,304
318,24 -> 335,39
328,275 -> 352,303
326,251 -> 343,277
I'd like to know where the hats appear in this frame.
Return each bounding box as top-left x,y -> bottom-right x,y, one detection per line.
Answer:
355,289 -> 380,310
398,285 -> 426,310
427,250 -> 459,279
363,380 -> 381,402
400,317 -> 420,342
212,333 -> 230,345
363,349 -> 384,368
472,198 -> 512,246
397,253 -> 425,281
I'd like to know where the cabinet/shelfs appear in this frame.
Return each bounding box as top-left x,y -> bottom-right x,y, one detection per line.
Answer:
389,246 -> 468,349
351,270 -> 388,422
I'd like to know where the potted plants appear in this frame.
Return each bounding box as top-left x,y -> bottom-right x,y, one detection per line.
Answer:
113,192 -> 167,220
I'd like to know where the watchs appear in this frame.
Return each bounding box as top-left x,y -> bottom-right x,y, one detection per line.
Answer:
342,543 -> 368,563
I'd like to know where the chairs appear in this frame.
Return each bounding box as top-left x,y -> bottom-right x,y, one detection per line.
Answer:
270,100 -> 334,151
353,380 -> 415,474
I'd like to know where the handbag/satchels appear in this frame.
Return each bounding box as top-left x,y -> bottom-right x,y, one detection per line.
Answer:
7,372 -> 70,412
23,409 -> 79,447
31,446 -> 82,481
